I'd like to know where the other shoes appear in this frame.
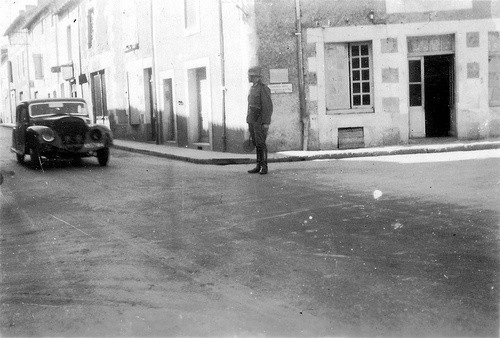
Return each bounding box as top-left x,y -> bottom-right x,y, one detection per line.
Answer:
259,166 -> 268,175
248,165 -> 261,173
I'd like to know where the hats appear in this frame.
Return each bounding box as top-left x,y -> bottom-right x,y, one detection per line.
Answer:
248,66 -> 262,77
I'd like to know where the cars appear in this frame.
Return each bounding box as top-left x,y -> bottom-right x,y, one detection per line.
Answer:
10,97 -> 115,170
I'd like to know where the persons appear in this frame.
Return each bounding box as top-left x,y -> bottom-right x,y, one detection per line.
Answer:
246,66 -> 273,175
